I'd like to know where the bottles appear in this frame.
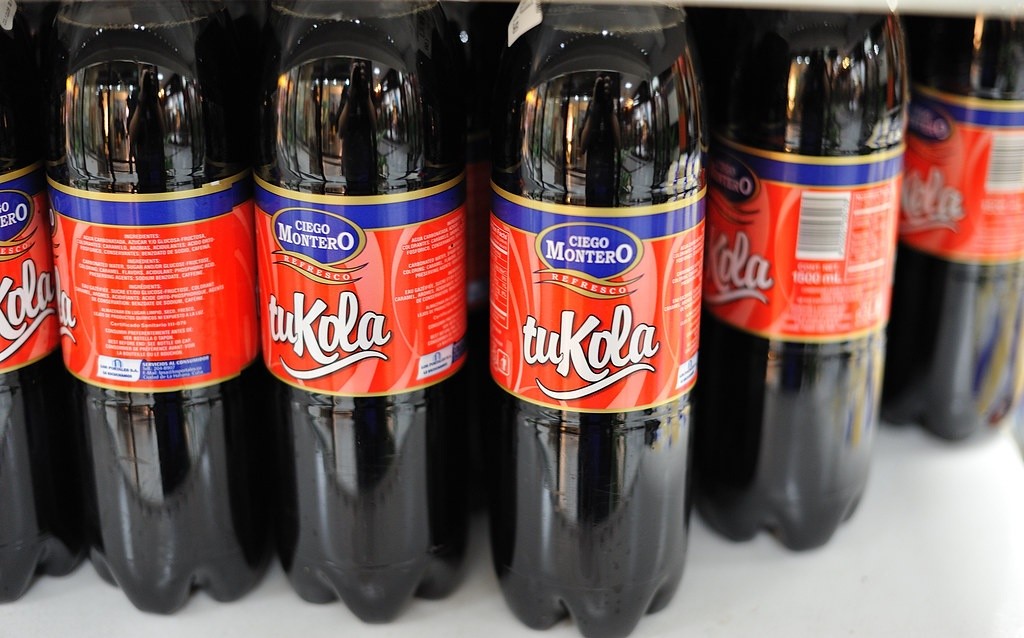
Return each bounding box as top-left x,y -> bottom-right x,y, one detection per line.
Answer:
253,0 -> 469,624
879,0 -> 1024,442
0,0 -> 91,604
491,0 -> 707,638
42,0 -> 278,614
696,0 -> 907,555
